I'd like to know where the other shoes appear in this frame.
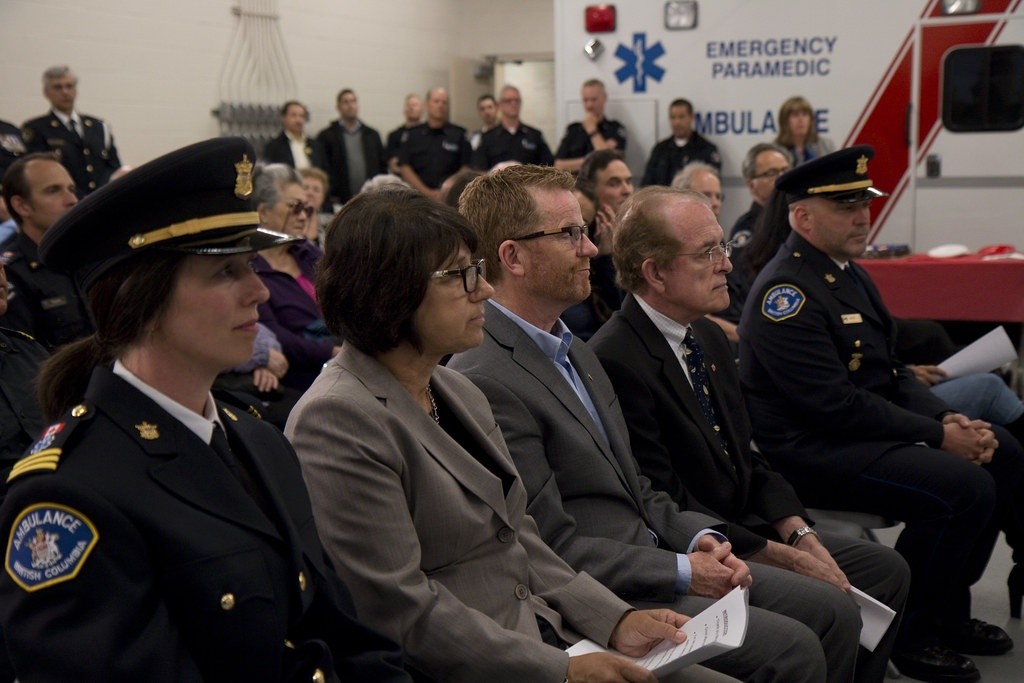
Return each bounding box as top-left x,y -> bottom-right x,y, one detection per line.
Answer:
1005,559 -> 1024,620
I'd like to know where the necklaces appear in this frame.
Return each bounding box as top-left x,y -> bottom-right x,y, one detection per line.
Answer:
426,385 -> 439,426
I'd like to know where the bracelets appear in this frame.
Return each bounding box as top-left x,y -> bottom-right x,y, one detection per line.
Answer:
588,131 -> 599,138
787,527 -> 823,548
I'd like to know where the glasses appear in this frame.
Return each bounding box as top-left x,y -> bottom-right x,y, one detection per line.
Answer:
503,97 -> 520,102
428,259 -> 489,294
294,202 -> 314,218
752,164 -> 793,180
509,221 -> 595,247
655,238 -> 737,265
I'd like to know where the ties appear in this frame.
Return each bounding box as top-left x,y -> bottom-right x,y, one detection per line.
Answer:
844,264 -> 870,304
677,327 -> 740,473
211,420 -> 260,506
68,118 -> 79,136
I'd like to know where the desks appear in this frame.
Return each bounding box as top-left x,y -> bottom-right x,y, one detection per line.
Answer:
851,252 -> 1024,323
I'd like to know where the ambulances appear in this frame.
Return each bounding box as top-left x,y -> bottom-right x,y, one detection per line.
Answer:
554,0 -> 1024,324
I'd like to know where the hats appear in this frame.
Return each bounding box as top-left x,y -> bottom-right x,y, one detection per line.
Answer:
776,144 -> 889,206
37,135 -> 308,304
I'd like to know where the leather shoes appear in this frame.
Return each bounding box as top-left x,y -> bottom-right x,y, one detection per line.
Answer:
890,636 -> 982,683
933,611 -> 1014,656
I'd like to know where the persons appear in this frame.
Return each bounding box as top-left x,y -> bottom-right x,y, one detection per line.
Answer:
0,67 -> 121,245
671,162 -> 751,362
221,163 -> 337,423
447,161 -> 864,683
283,188 -> 744,683
551,80 -> 627,173
0,257 -> 58,468
560,150 -> 633,341
585,185 -> 910,683
737,145 -> 1024,683
0,151 -> 97,341
0,136 -> 448,683
265,83 -> 555,208
728,142 -> 795,266
641,98 -> 723,186
776,96 -> 833,166
